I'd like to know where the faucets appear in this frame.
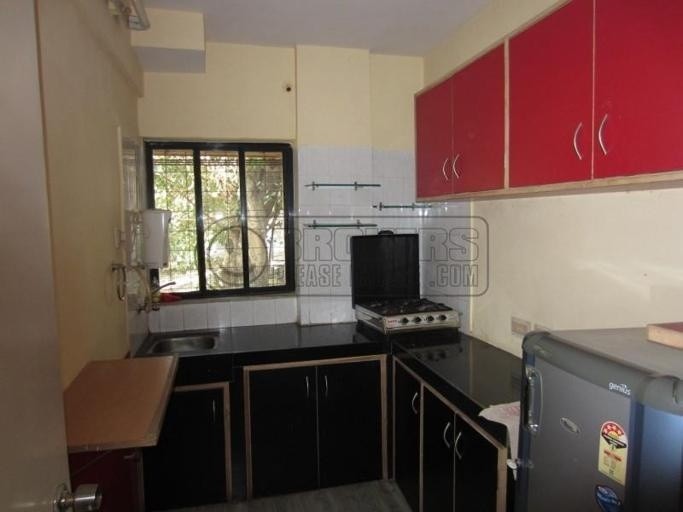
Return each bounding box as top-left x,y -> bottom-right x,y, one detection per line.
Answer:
144,281 -> 176,310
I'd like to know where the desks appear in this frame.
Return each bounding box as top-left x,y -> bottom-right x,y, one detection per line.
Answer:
61,354 -> 179,512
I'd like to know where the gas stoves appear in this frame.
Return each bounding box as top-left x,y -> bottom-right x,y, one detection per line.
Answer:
352,297 -> 460,330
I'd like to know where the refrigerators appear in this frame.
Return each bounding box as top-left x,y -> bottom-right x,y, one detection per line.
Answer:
510,318 -> 683,512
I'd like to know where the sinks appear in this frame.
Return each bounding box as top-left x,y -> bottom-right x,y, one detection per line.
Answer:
142,331 -> 219,354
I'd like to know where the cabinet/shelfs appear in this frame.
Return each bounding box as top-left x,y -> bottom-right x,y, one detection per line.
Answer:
424,379 -> 510,512
146,384 -> 233,507
512,2 -> 683,184
390,352 -> 424,512
242,356 -> 389,502
413,38 -> 507,201
